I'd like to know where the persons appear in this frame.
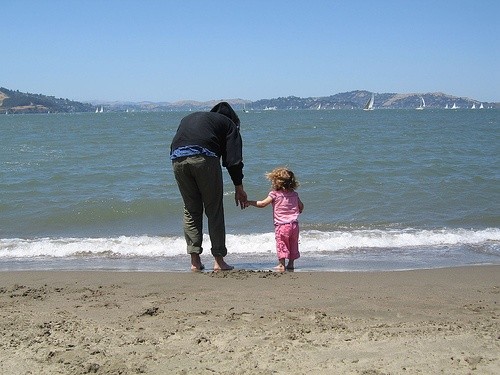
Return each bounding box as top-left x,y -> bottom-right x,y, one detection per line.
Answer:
246,166 -> 305,271
169,101 -> 247,272
